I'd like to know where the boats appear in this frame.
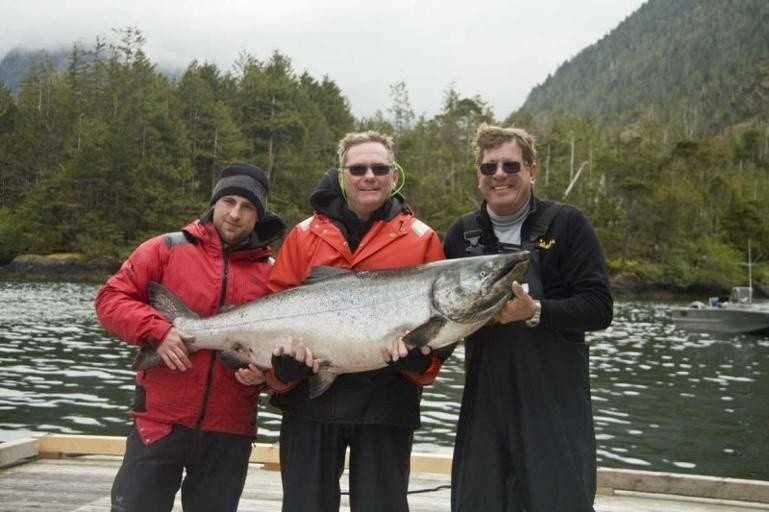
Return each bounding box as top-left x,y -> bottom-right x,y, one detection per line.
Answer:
664,299 -> 769,335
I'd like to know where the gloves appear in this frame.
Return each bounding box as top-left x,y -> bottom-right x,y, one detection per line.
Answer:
270,350 -> 319,385
386,344 -> 435,375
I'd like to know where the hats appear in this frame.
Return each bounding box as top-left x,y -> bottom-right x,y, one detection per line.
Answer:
210,162 -> 270,220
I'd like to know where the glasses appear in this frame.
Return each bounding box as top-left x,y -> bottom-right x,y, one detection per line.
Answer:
476,162 -> 530,176
339,164 -> 391,178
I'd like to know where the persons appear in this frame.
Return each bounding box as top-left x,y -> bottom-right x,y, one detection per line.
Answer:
266,130 -> 444,512
434,126 -> 614,512
94,162 -> 287,512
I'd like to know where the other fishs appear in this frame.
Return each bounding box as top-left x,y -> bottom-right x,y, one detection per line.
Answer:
131,247 -> 537,399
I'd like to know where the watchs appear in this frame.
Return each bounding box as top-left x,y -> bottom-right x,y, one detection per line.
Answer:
525,300 -> 542,328
